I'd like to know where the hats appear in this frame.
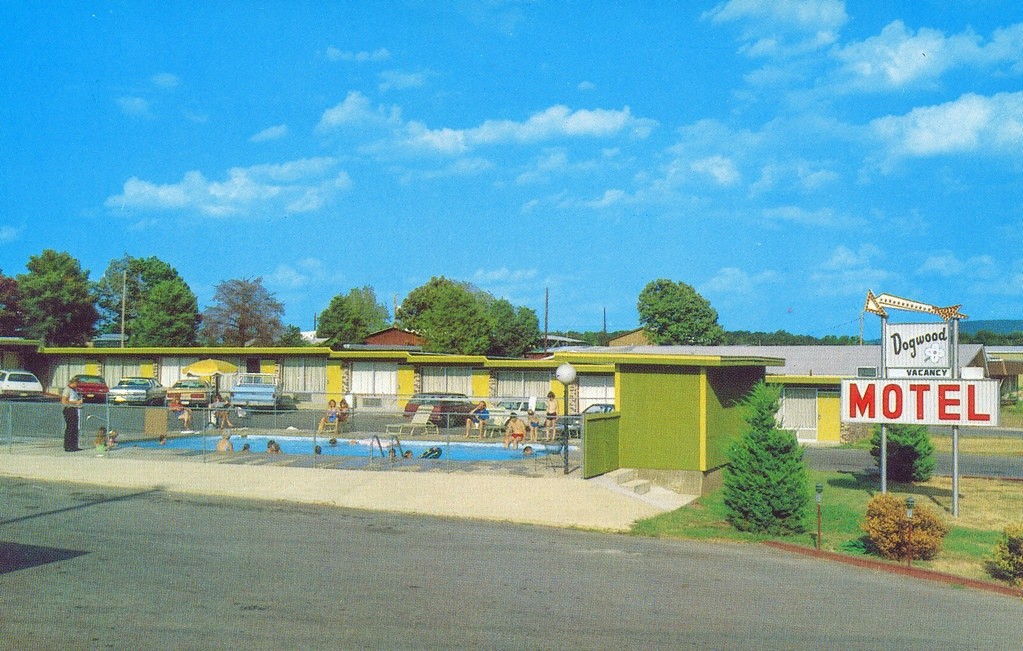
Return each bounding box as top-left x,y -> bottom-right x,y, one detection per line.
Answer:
67,377 -> 81,383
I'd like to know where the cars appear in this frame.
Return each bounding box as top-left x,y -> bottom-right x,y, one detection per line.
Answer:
0,370 -> 42,398
403,392 -> 476,427
166,379 -> 215,407
69,374 -> 109,403
108,376 -> 167,406
557,404 -> 615,428
488,400 -> 548,427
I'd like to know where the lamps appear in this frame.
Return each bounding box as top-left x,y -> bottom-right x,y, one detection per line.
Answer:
815,483 -> 823,504
905,495 -> 914,518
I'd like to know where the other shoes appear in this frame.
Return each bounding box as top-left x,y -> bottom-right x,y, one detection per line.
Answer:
65,448 -> 82,451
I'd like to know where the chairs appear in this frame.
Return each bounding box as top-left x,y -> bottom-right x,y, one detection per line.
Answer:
535,435 -> 565,472
205,406 -> 226,429
238,406 -> 256,429
385,404 -> 439,435
318,411 -> 347,435
468,413 -> 504,438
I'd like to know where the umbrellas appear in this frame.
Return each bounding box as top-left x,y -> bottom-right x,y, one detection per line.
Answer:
180,357 -> 239,404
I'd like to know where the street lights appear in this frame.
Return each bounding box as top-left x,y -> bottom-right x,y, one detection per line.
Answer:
556,365 -> 575,474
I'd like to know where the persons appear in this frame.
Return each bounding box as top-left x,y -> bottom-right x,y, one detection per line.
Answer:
216,428 -> 283,454
316,398 -> 350,435
544,391 -> 559,442
315,438 -> 337,454
464,401 -> 489,440
167,396 -> 192,431
527,409 -> 540,443
94,426 -> 118,447
389,448 -> 413,459
208,393 -> 234,428
62,377 -> 84,452
523,446 -> 532,455
158,435 -> 167,445
504,412 -> 527,450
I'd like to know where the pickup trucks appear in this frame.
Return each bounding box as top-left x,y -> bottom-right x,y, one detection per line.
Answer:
229,373 -> 284,408
